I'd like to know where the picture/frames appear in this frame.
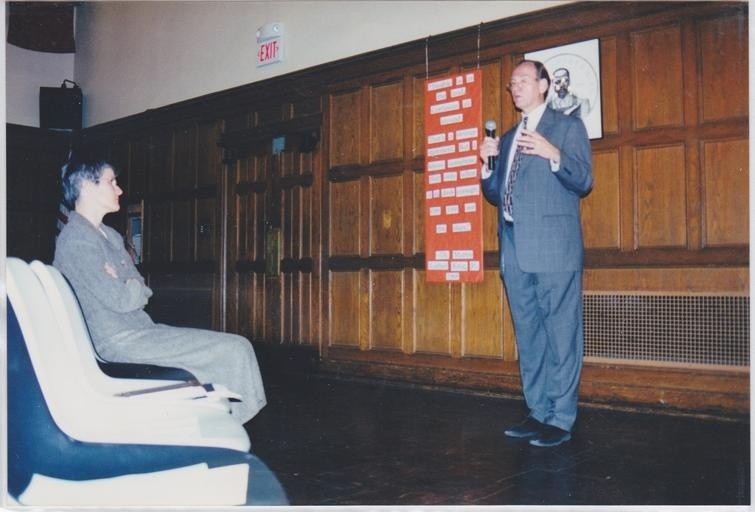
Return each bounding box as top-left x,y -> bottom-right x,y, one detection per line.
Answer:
523,38 -> 603,140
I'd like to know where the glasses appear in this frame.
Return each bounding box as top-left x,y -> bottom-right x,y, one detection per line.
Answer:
505,77 -> 533,90
89,175 -> 119,185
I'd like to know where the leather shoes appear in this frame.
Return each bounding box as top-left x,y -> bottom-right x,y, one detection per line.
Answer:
527,425 -> 573,448
503,417 -> 539,439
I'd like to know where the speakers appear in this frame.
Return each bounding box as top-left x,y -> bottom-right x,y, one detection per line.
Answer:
39,81 -> 81,134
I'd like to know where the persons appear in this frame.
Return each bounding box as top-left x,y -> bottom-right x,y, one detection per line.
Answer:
478,60 -> 595,448
54,147 -> 268,426
548,68 -> 591,116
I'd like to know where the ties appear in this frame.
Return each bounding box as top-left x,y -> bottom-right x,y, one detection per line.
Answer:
502,117 -> 529,217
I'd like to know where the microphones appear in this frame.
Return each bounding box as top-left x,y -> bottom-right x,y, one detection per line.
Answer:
486,120 -> 497,173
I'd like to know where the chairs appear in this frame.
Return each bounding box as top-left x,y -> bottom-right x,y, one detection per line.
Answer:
6,256 -> 290,505
29,260 -> 207,399
7,258 -> 251,452
